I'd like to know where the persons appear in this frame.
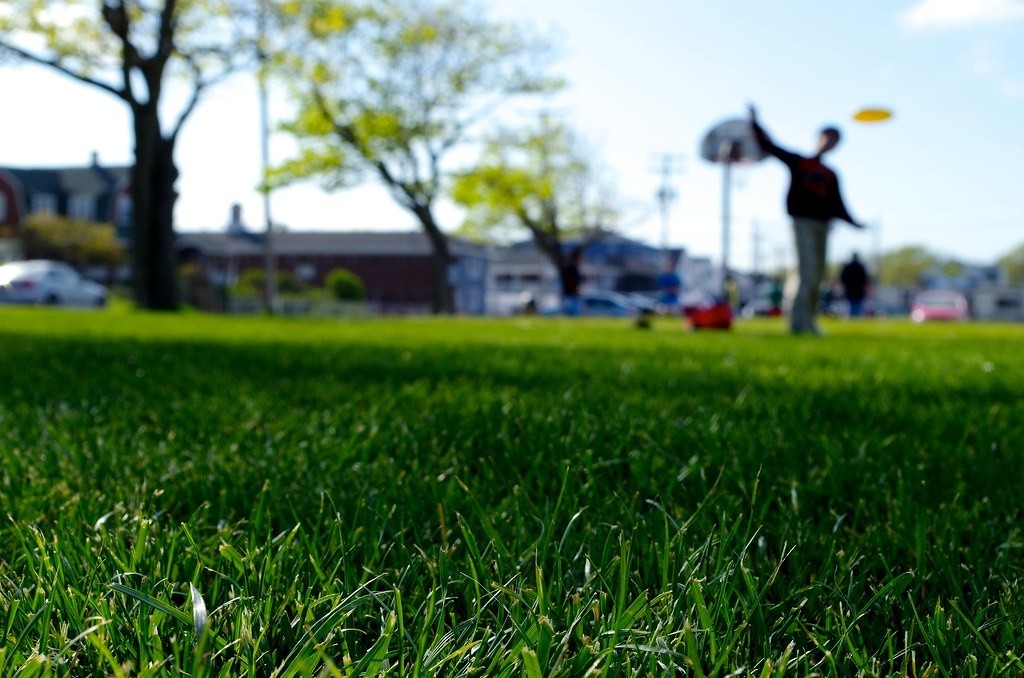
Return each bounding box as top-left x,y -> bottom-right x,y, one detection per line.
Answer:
747,105 -> 866,338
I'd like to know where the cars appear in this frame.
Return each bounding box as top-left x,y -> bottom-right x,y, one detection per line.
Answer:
912,290 -> 970,323
0,258 -> 109,306
579,290 -> 639,315
